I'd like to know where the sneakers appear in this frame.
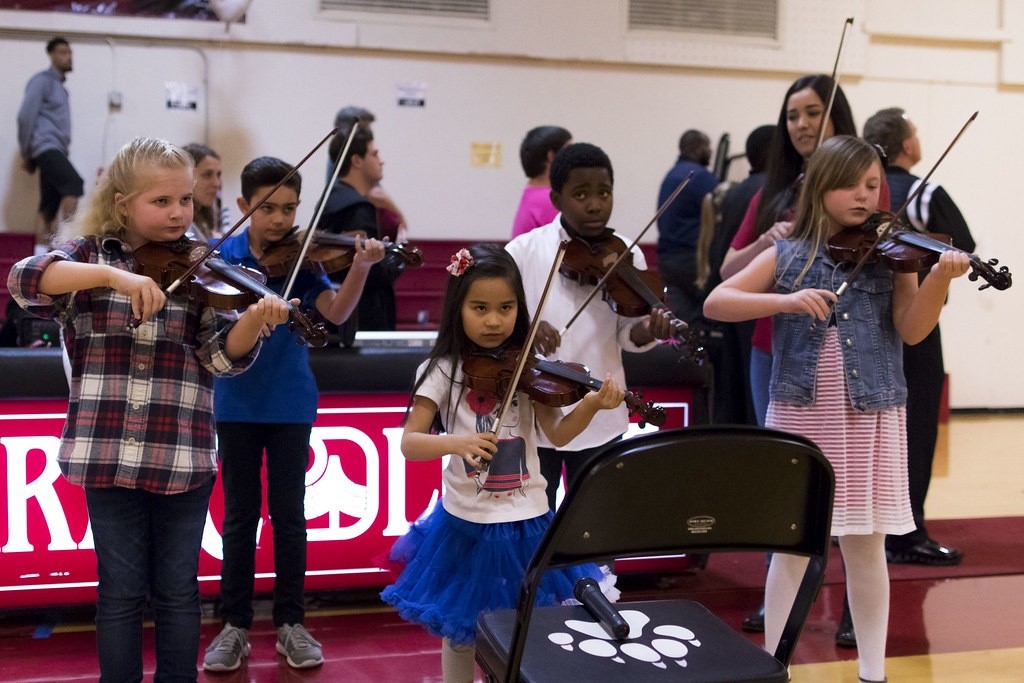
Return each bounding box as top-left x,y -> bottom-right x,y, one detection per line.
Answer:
203,625 -> 254,673
275,624 -> 325,668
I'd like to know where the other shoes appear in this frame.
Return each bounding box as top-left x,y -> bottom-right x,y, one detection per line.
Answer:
742,604 -> 767,630
835,597 -> 858,646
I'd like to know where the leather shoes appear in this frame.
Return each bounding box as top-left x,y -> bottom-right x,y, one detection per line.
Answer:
886,538 -> 964,567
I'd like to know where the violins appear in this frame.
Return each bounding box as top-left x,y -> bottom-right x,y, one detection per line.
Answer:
460,343 -> 669,428
825,210 -> 1013,292
254,229 -> 427,278
133,236 -> 331,351
556,231 -> 710,370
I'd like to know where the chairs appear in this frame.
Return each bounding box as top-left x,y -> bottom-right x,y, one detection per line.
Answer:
471,422 -> 837,683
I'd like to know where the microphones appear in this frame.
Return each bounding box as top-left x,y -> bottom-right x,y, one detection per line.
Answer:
572,577 -> 630,639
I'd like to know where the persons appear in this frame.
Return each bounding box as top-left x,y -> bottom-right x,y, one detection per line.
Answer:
179,107 -> 407,331
655,108 -> 976,565
18,36 -> 84,256
380,142 -> 689,683
720,74 -> 891,649
8,138 -> 388,683
511,125 -> 573,239
702,134 -> 968,683
